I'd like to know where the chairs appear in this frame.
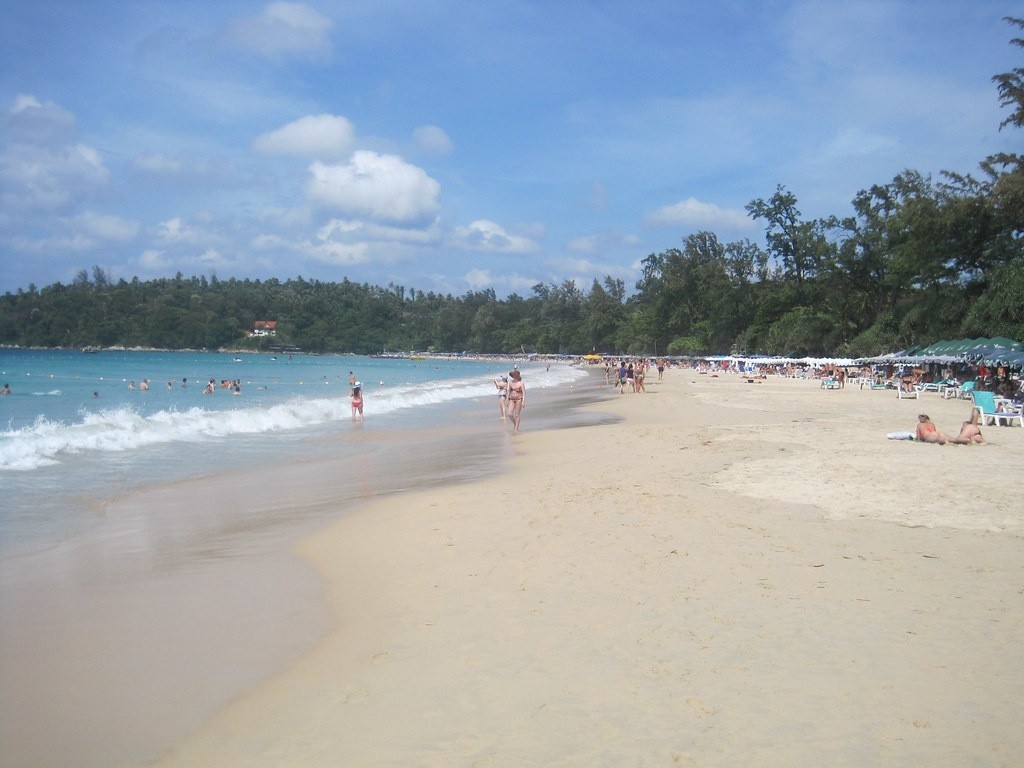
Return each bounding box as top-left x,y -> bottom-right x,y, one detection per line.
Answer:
696,366 -> 1024,427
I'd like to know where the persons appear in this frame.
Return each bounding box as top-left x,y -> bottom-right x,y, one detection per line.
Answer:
436,365 -> 438,373
0,383 -> 11,395
667,359 -> 849,389
337,375 -> 341,380
128,379 -> 151,391
350,382 -> 364,423
948,421 -> 984,444
603,357 -> 651,393
167,382 -> 172,391
203,378 -> 242,395
288,356 -> 293,360
505,371 -> 526,432
988,402 -> 1008,426
348,372 -> 357,398
182,378 -> 187,388
656,356 -> 665,381
493,374 -> 508,420
916,414 -> 954,445
431,355 -> 581,371
90,391 -> 100,399
321,376 -> 328,382
265,386 -> 267,389
858,368 -> 1014,400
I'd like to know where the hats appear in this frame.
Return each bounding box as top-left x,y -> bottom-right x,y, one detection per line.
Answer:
353,381 -> 361,389
509,370 -> 522,381
501,375 -> 508,383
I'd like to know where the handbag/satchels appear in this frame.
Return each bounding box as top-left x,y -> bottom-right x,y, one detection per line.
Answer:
620,378 -> 626,384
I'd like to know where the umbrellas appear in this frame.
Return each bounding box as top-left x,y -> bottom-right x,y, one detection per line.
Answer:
981,349 -> 1024,369
855,336 -> 1024,365
